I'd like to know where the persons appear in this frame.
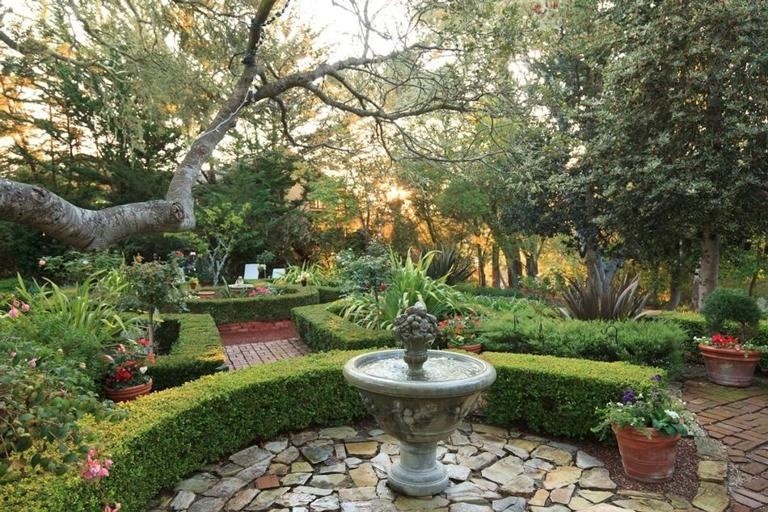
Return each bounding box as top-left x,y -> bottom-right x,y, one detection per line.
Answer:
235,275 -> 245,285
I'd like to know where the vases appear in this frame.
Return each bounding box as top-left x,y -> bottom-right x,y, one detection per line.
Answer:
611,420 -> 683,482
447,343 -> 481,354
103,378 -> 155,403
700,346 -> 760,386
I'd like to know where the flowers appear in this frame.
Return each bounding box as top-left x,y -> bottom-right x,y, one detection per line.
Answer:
590,375 -> 708,445
437,317 -> 487,348
695,333 -> 767,358
103,339 -> 160,392
